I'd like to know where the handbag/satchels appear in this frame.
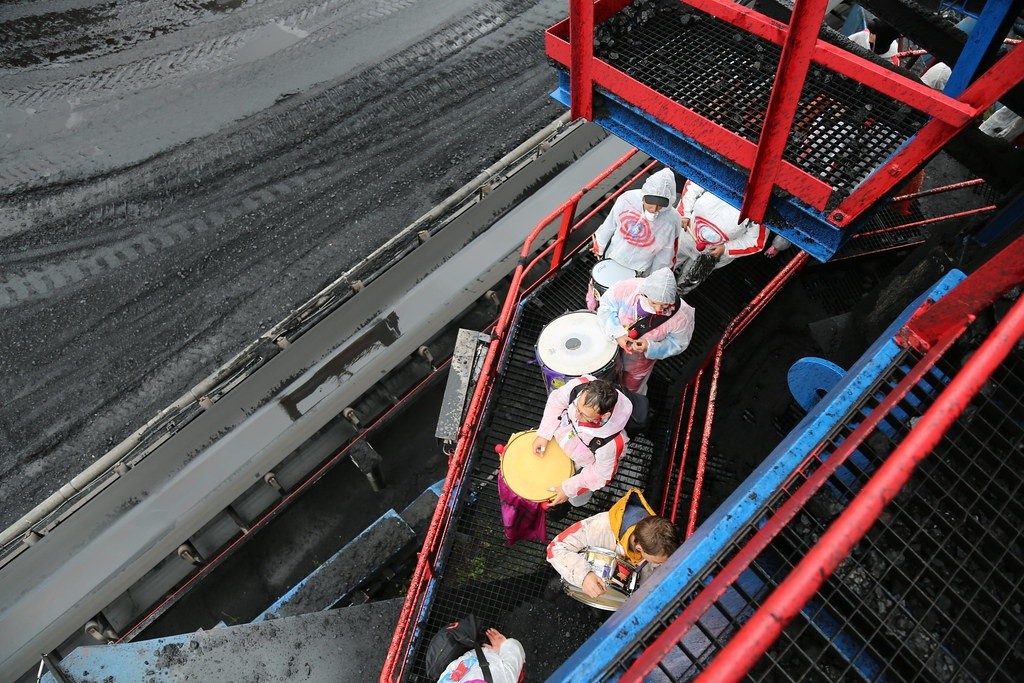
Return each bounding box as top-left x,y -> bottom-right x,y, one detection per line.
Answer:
425,614 -> 485,681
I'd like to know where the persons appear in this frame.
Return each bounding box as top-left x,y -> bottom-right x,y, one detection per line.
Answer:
436,627 -> 526,683
590,167 -> 793,397
847,16 -> 953,91
546,488 -> 682,598
532,375 -> 633,522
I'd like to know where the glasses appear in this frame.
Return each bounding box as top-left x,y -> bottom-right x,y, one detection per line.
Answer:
572,394 -> 601,423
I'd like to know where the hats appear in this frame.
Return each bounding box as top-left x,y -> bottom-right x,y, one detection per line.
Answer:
644,195 -> 669,207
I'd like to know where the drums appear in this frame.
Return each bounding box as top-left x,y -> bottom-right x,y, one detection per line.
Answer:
533,309 -> 623,399
587,258 -> 640,309
560,546 -> 643,612
499,428 -> 576,504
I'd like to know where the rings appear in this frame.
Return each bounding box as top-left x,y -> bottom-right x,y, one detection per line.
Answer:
550,487 -> 555,492
541,446 -> 546,451
